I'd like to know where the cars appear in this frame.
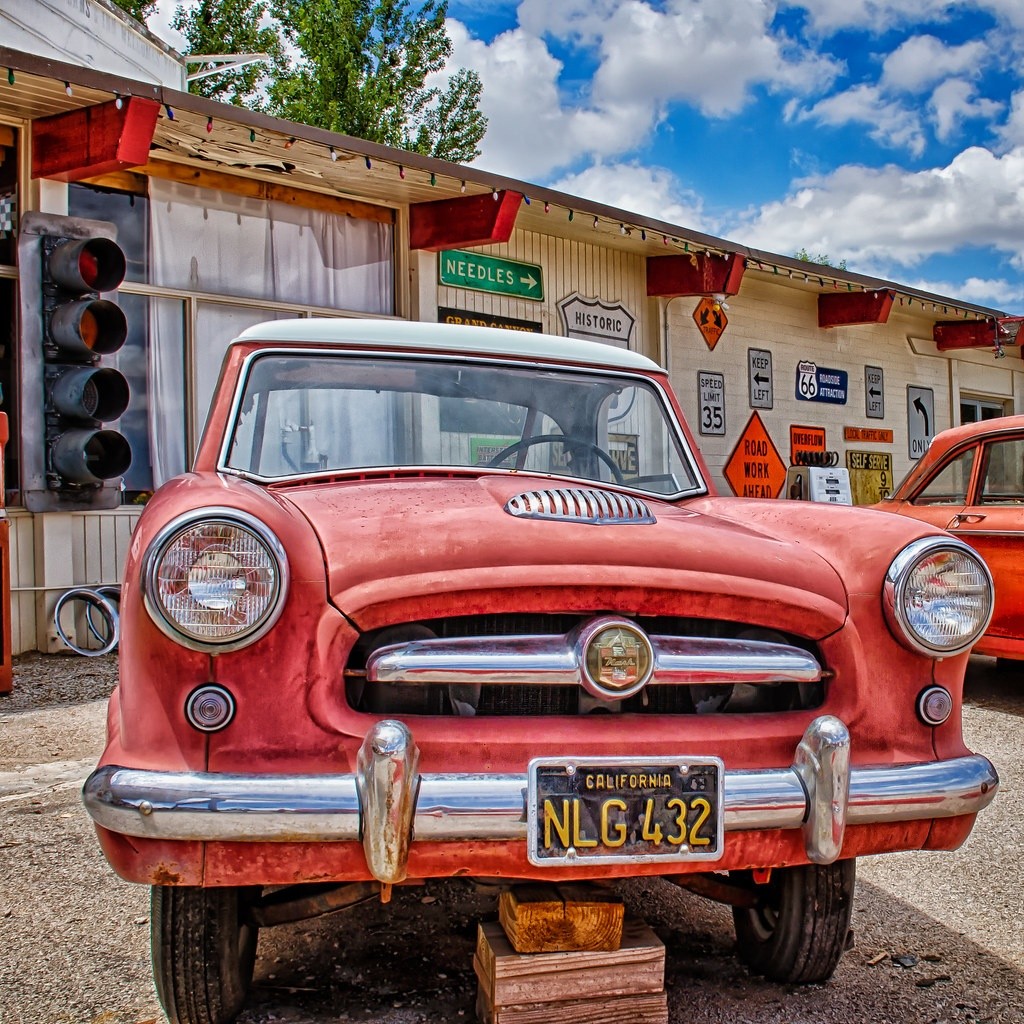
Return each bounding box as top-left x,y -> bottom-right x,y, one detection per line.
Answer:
862,415 -> 1023,666
82,316 -> 1001,1024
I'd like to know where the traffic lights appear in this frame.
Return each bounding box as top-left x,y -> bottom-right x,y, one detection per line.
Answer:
16,212 -> 131,514
